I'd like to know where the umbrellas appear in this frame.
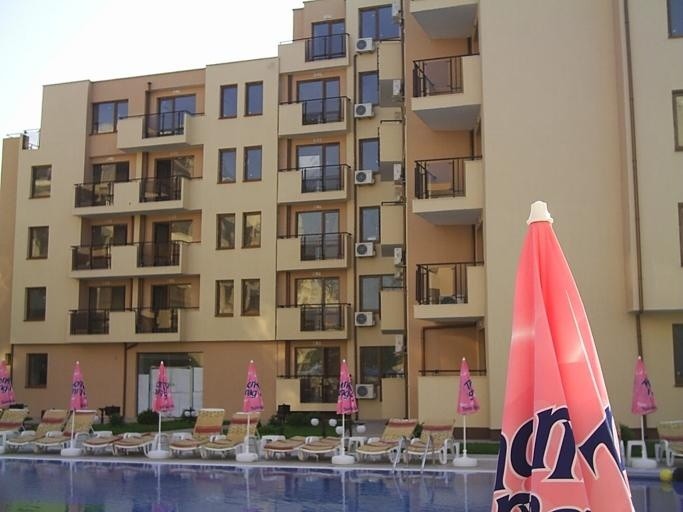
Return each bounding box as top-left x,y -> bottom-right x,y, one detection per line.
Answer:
631,355 -> 657,459
337,359 -> 359,455
491,201 -> 636,512
0,360 -> 16,409
152,361 -> 172,450
456,357 -> 480,457
67,361 -> 88,447
243,360 -> 264,452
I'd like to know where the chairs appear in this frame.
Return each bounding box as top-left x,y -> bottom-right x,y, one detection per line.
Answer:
170,408 -> 225,458
199,411 -> 260,460
32,410 -> 97,453
265,435 -> 306,461
6,409 -> 68,454
301,436 -> 346,460
355,417 -> 418,473
651,419 -> 682,466
113,433 -> 157,456
1,409 -> 30,452
404,416 -> 459,474
82,432 -> 123,456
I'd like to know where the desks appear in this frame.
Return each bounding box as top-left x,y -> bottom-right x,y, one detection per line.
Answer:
262,435 -> 286,460
348,436 -> 369,460
171,432 -> 192,441
92,431 -> 112,439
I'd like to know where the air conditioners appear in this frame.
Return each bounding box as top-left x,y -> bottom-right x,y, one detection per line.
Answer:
392,247 -> 403,267
392,163 -> 401,181
353,311 -> 375,327
354,383 -> 376,400
354,242 -> 375,257
351,1 -> 403,119
438,294 -> 465,305
353,169 -> 374,185
